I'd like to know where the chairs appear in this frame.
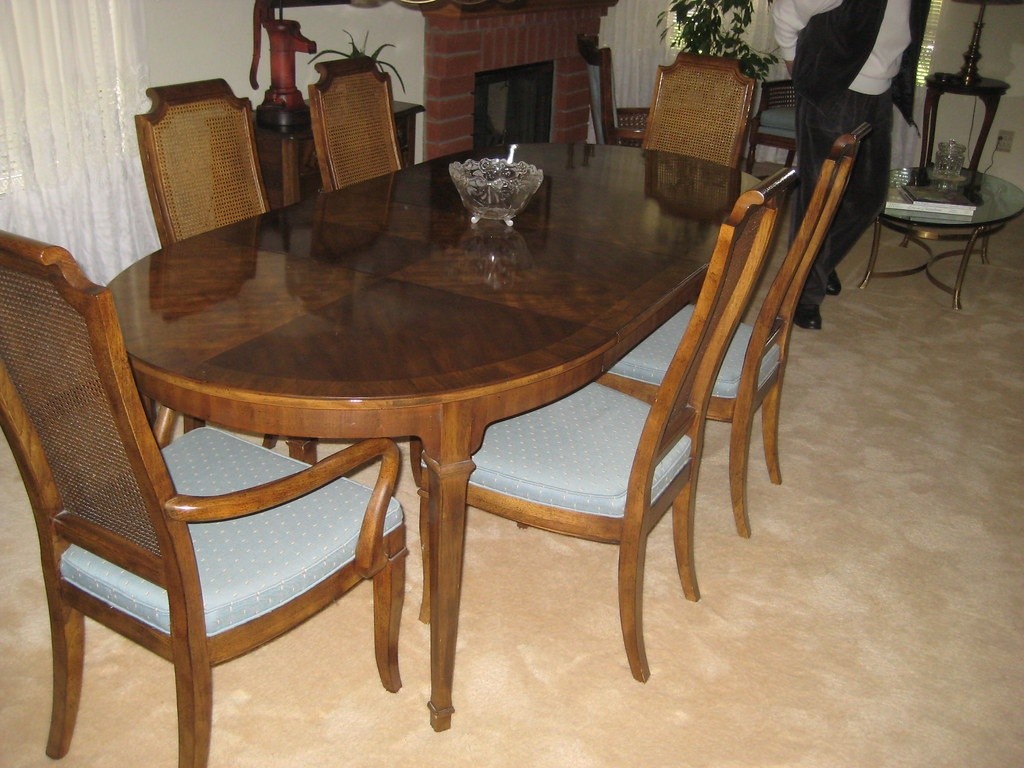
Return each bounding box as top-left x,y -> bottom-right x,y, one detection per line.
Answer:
577,32 -> 649,148
416,166 -> 801,684
1,228 -> 412,767
132,76 -> 281,449
308,57 -> 406,192
643,52 -> 761,168
515,120 -> 873,542
746,78 -> 798,175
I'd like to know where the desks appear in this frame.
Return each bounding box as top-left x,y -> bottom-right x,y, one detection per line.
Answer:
919,74 -> 1011,170
859,166 -> 1024,311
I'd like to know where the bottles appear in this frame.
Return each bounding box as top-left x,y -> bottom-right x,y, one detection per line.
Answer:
936,140 -> 966,177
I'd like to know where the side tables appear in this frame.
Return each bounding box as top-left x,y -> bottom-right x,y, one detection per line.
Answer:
251,95 -> 427,210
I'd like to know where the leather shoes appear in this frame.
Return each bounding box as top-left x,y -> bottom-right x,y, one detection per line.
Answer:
825,269 -> 841,295
793,303 -> 821,329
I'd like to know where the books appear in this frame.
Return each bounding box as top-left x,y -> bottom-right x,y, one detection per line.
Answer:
886,186 -> 976,217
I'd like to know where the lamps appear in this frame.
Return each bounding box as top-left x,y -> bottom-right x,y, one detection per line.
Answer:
951,0 -> 1024,82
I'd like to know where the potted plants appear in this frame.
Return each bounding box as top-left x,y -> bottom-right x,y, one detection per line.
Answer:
656,0 -> 778,119
309,28 -> 406,100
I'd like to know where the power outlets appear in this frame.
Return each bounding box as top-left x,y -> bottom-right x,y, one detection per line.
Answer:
996,129 -> 1014,153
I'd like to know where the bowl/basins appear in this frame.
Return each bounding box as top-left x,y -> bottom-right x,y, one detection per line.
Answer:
448,159 -> 544,220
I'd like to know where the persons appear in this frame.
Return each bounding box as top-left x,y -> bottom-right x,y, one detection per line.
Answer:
768,0 -> 925,330
